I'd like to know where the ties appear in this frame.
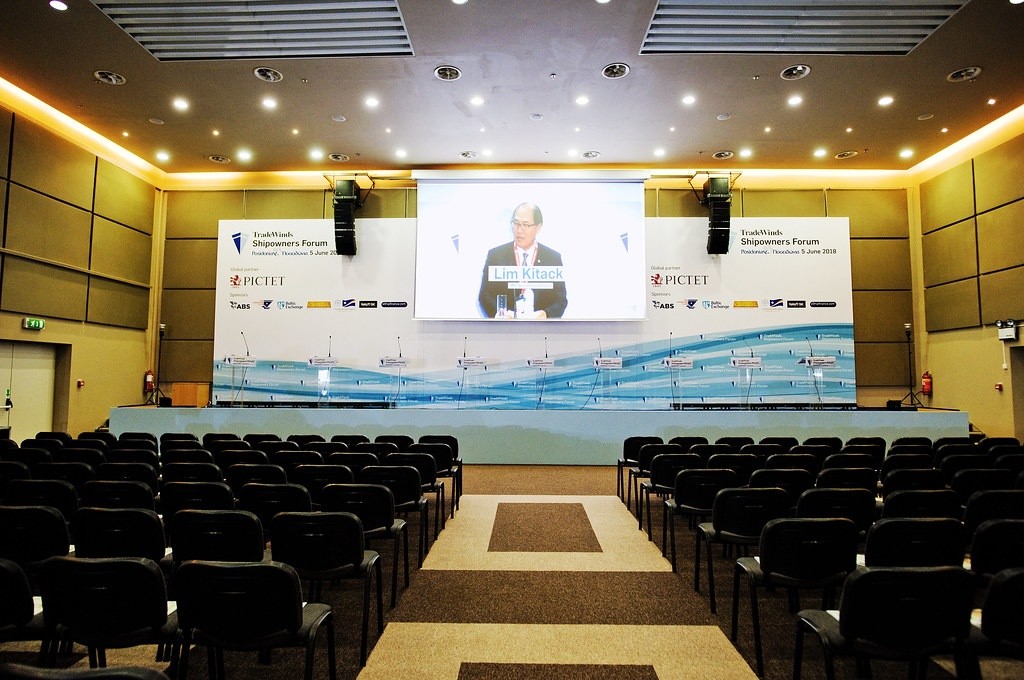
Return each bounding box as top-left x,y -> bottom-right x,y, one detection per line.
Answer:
522,253 -> 528,266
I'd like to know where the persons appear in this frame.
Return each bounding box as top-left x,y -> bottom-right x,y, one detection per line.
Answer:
479,202 -> 568,320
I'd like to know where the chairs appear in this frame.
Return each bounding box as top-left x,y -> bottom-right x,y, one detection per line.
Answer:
615,436 -> 1024,680
0,430 -> 462,680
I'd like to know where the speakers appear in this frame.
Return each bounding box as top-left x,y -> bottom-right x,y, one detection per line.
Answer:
702,178 -> 729,198
707,201 -> 731,255
334,202 -> 356,255
335,180 -> 360,201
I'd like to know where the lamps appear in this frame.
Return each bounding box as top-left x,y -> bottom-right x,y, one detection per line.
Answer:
996,318 -> 1017,340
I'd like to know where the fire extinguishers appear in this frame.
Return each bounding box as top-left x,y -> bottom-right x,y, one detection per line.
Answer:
145,369 -> 154,391
922,371 -> 932,395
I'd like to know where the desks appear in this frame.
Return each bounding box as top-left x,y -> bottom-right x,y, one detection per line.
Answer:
110,407 -> 969,467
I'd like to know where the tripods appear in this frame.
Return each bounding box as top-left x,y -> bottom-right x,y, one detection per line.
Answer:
900,330 -> 924,408
146,336 -> 165,406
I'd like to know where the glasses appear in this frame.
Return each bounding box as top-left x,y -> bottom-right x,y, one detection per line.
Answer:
511,220 -> 537,230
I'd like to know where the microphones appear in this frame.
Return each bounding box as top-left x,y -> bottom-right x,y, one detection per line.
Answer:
545,337 -> 547,358
598,338 -> 602,358
241,332 -> 249,356
398,337 -> 401,357
806,337 -> 812,357
743,337 -> 753,357
328,336 -> 332,357
463,337 -> 467,357
669,332 -> 672,358
